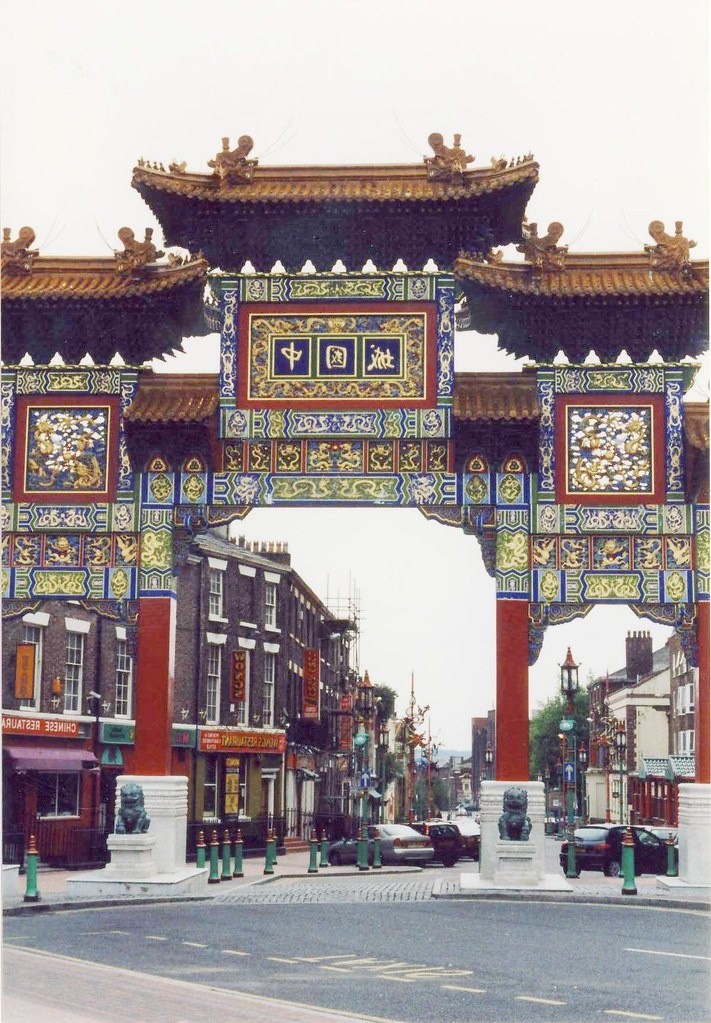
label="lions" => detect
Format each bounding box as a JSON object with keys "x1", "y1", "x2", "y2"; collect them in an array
[
  {"x1": 497, "y1": 786, "x2": 533, "y2": 841},
  {"x1": 116, "y1": 783, "x2": 151, "y2": 834}
]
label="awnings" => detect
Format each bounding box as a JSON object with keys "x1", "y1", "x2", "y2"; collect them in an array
[
  {"x1": 2, "y1": 746, "x2": 101, "y2": 776},
  {"x1": 303, "y1": 768, "x2": 321, "y2": 782},
  {"x1": 369, "y1": 790, "x2": 382, "y2": 799}
]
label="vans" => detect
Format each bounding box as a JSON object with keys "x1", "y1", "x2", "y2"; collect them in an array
[{"x1": 406, "y1": 823, "x2": 463, "y2": 867}]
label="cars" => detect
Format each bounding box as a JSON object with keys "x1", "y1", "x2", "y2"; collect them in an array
[
  {"x1": 642, "y1": 826, "x2": 680, "y2": 846},
  {"x1": 455, "y1": 803, "x2": 479, "y2": 818},
  {"x1": 327, "y1": 824, "x2": 435, "y2": 868},
  {"x1": 559, "y1": 824, "x2": 679, "y2": 877},
  {"x1": 442, "y1": 818, "x2": 480, "y2": 861}
]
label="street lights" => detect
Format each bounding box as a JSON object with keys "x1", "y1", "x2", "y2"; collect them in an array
[
  {"x1": 357, "y1": 670, "x2": 374, "y2": 871},
  {"x1": 557, "y1": 647, "x2": 582, "y2": 879}
]
